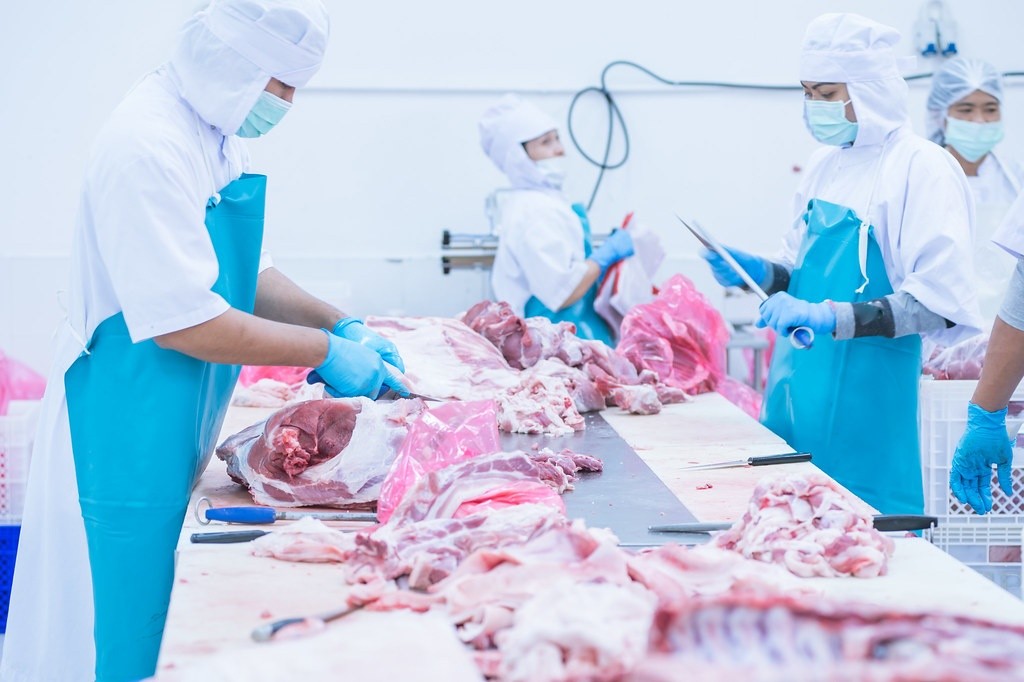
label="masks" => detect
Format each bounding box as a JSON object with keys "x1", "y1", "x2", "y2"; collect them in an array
[
  {"x1": 535, "y1": 158, "x2": 571, "y2": 193},
  {"x1": 801, "y1": 100, "x2": 858, "y2": 149},
  {"x1": 236, "y1": 90, "x2": 294, "y2": 139},
  {"x1": 943, "y1": 116, "x2": 1005, "y2": 164}
]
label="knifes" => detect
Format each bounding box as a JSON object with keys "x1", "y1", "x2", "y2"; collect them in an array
[
  {"x1": 682, "y1": 452, "x2": 815, "y2": 471},
  {"x1": 306, "y1": 369, "x2": 442, "y2": 404}
]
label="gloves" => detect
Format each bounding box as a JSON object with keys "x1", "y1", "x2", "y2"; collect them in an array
[
  {"x1": 587, "y1": 227, "x2": 634, "y2": 286},
  {"x1": 753, "y1": 291, "x2": 836, "y2": 338},
  {"x1": 949, "y1": 400, "x2": 1014, "y2": 516},
  {"x1": 702, "y1": 245, "x2": 771, "y2": 288},
  {"x1": 306, "y1": 316, "x2": 413, "y2": 401}
]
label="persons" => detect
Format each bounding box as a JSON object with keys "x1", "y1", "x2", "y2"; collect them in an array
[
  {"x1": 479, "y1": 93, "x2": 634, "y2": 350},
  {"x1": 703, "y1": 11, "x2": 1024, "y2": 539},
  {"x1": 0, "y1": 0, "x2": 411, "y2": 682}
]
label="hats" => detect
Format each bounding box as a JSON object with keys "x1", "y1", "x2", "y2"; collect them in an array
[
  {"x1": 925, "y1": 51, "x2": 1004, "y2": 146},
  {"x1": 795, "y1": 12, "x2": 913, "y2": 147},
  {"x1": 475, "y1": 91, "x2": 561, "y2": 193},
  {"x1": 164, "y1": 0, "x2": 334, "y2": 136}
]
